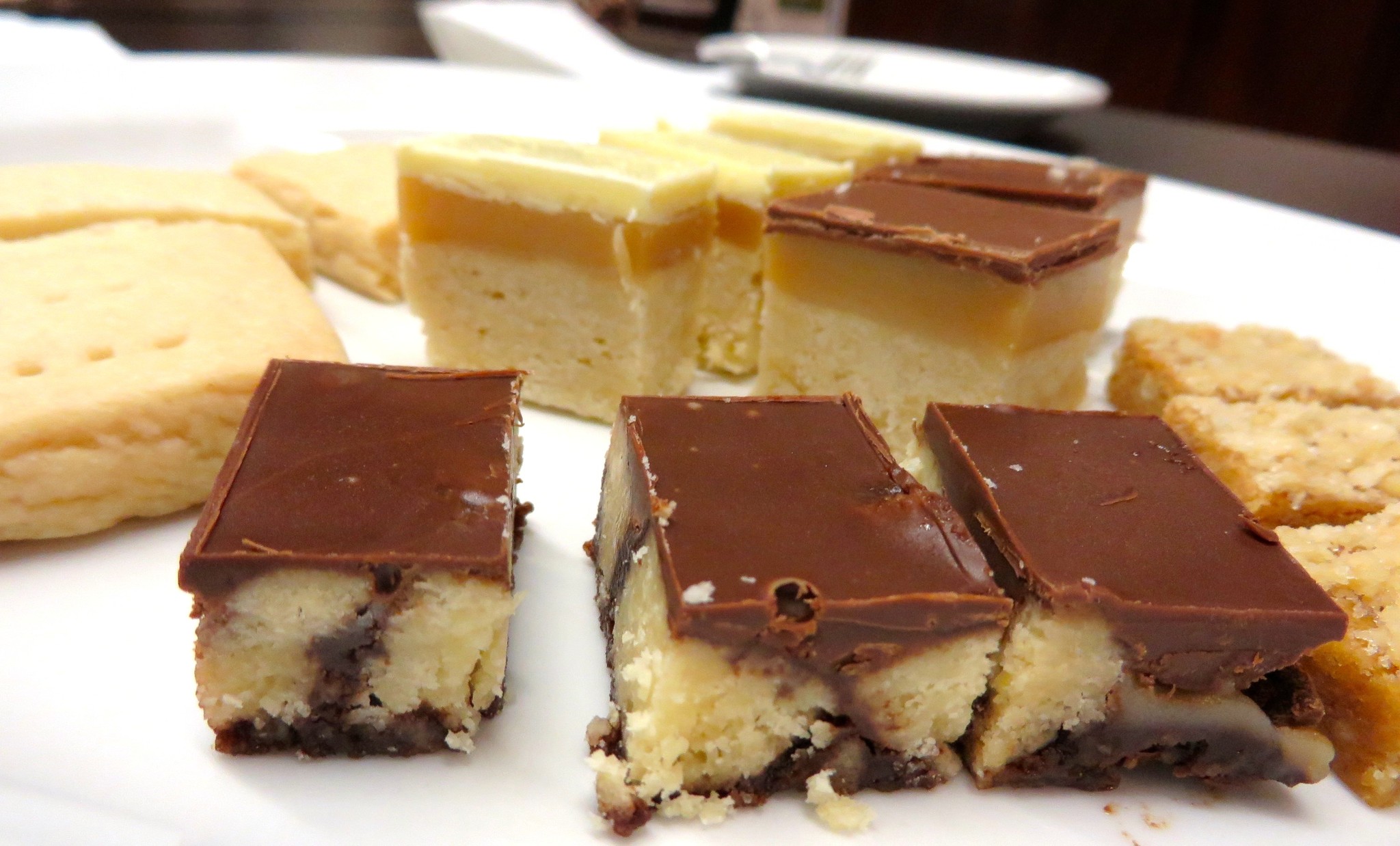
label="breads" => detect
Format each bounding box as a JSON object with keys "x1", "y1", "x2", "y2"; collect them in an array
[{"x1": 0, "y1": 146, "x2": 400, "y2": 542}]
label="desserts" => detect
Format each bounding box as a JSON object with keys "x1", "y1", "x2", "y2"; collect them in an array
[
  {"x1": 913, "y1": 400, "x2": 1349, "y2": 790},
  {"x1": 1106, "y1": 321, "x2": 1399, "y2": 810},
  {"x1": 584, "y1": 398, "x2": 1016, "y2": 838},
  {"x1": 758, "y1": 156, "x2": 1146, "y2": 470},
  {"x1": 393, "y1": 113, "x2": 922, "y2": 426},
  {"x1": 181, "y1": 360, "x2": 532, "y2": 759}
]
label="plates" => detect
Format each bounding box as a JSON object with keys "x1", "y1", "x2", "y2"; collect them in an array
[
  {"x1": 0, "y1": 52, "x2": 1397, "y2": 843},
  {"x1": 694, "y1": 30, "x2": 1113, "y2": 130}
]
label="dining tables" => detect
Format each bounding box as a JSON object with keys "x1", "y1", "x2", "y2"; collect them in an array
[{"x1": 1, "y1": 23, "x2": 1400, "y2": 236}]
